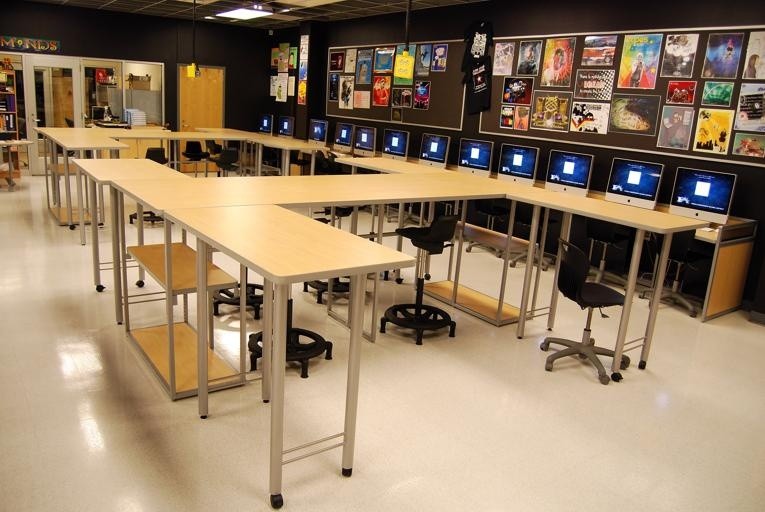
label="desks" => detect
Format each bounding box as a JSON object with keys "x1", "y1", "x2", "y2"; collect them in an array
[
  {"x1": 72, "y1": 158, "x2": 193, "y2": 291},
  {"x1": 334, "y1": 157, "x2": 558, "y2": 328},
  {"x1": 38, "y1": 127, "x2": 130, "y2": 230},
  {"x1": 0, "y1": 139, "x2": 34, "y2": 191},
  {"x1": 165, "y1": 206, "x2": 418, "y2": 511},
  {"x1": 195, "y1": 128, "x2": 272, "y2": 177},
  {"x1": 32, "y1": 126, "x2": 170, "y2": 227},
  {"x1": 170, "y1": 131, "x2": 276, "y2": 178},
  {"x1": 111, "y1": 169, "x2": 508, "y2": 403},
  {"x1": 246, "y1": 137, "x2": 331, "y2": 175},
  {"x1": 508, "y1": 191, "x2": 711, "y2": 382}
]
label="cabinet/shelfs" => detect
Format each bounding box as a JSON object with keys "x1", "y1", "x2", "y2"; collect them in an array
[{"x1": 0, "y1": 68, "x2": 21, "y2": 180}]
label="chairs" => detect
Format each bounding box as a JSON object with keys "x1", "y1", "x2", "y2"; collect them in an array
[
  {"x1": 315, "y1": 150, "x2": 328, "y2": 172},
  {"x1": 380, "y1": 214, "x2": 457, "y2": 344},
  {"x1": 206, "y1": 140, "x2": 225, "y2": 177},
  {"x1": 326, "y1": 150, "x2": 344, "y2": 175},
  {"x1": 182, "y1": 140, "x2": 209, "y2": 178},
  {"x1": 510, "y1": 202, "x2": 548, "y2": 271},
  {"x1": 216, "y1": 148, "x2": 240, "y2": 177},
  {"x1": 358, "y1": 167, "x2": 392, "y2": 216},
  {"x1": 388, "y1": 203, "x2": 425, "y2": 228},
  {"x1": 324, "y1": 205, "x2": 368, "y2": 229},
  {"x1": 303, "y1": 217, "x2": 349, "y2": 304},
  {"x1": 17, "y1": 117, "x2": 28, "y2": 168},
  {"x1": 248, "y1": 282, "x2": 333, "y2": 378},
  {"x1": 228, "y1": 140, "x2": 240, "y2": 150},
  {"x1": 214, "y1": 266, "x2": 264, "y2": 320},
  {"x1": 639, "y1": 229, "x2": 698, "y2": 320},
  {"x1": 586, "y1": 217, "x2": 629, "y2": 287},
  {"x1": 64, "y1": 118, "x2": 75, "y2": 159},
  {"x1": 129, "y1": 209, "x2": 163, "y2": 224},
  {"x1": 465, "y1": 198, "x2": 511, "y2": 259},
  {"x1": 145, "y1": 147, "x2": 168, "y2": 166},
  {"x1": 539, "y1": 237, "x2": 630, "y2": 384},
  {"x1": 440, "y1": 201, "x2": 461, "y2": 216},
  {"x1": 583, "y1": 230, "x2": 599, "y2": 262},
  {"x1": 313, "y1": 205, "x2": 364, "y2": 227},
  {"x1": 640, "y1": 243, "x2": 671, "y2": 289}
]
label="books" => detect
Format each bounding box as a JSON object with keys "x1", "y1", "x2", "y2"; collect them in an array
[
  {"x1": 0, "y1": 113, "x2": 14, "y2": 130},
  {"x1": 125, "y1": 108, "x2": 146, "y2": 126},
  {"x1": 0, "y1": 93, "x2": 15, "y2": 110}
]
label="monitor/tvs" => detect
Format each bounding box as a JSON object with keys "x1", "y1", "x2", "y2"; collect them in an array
[
  {"x1": 382, "y1": 129, "x2": 410, "y2": 161},
  {"x1": 260, "y1": 115, "x2": 271, "y2": 132},
  {"x1": 544, "y1": 149, "x2": 594, "y2": 197},
  {"x1": 308, "y1": 119, "x2": 328, "y2": 146},
  {"x1": 419, "y1": 134, "x2": 451, "y2": 168},
  {"x1": 605, "y1": 157, "x2": 664, "y2": 209},
  {"x1": 278, "y1": 116, "x2": 289, "y2": 134},
  {"x1": 458, "y1": 138, "x2": 494, "y2": 175},
  {"x1": 669, "y1": 167, "x2": 736, "y2": 224},
  {"x1": 498, "y1": 143, "x2": 540, "y2": 184},
  {"x1": 333, "y1": 122, "x2": 354, "y2": 154},
  {"x1": 354, "y1": 126, "x2": 376, "y2": 156}
]
